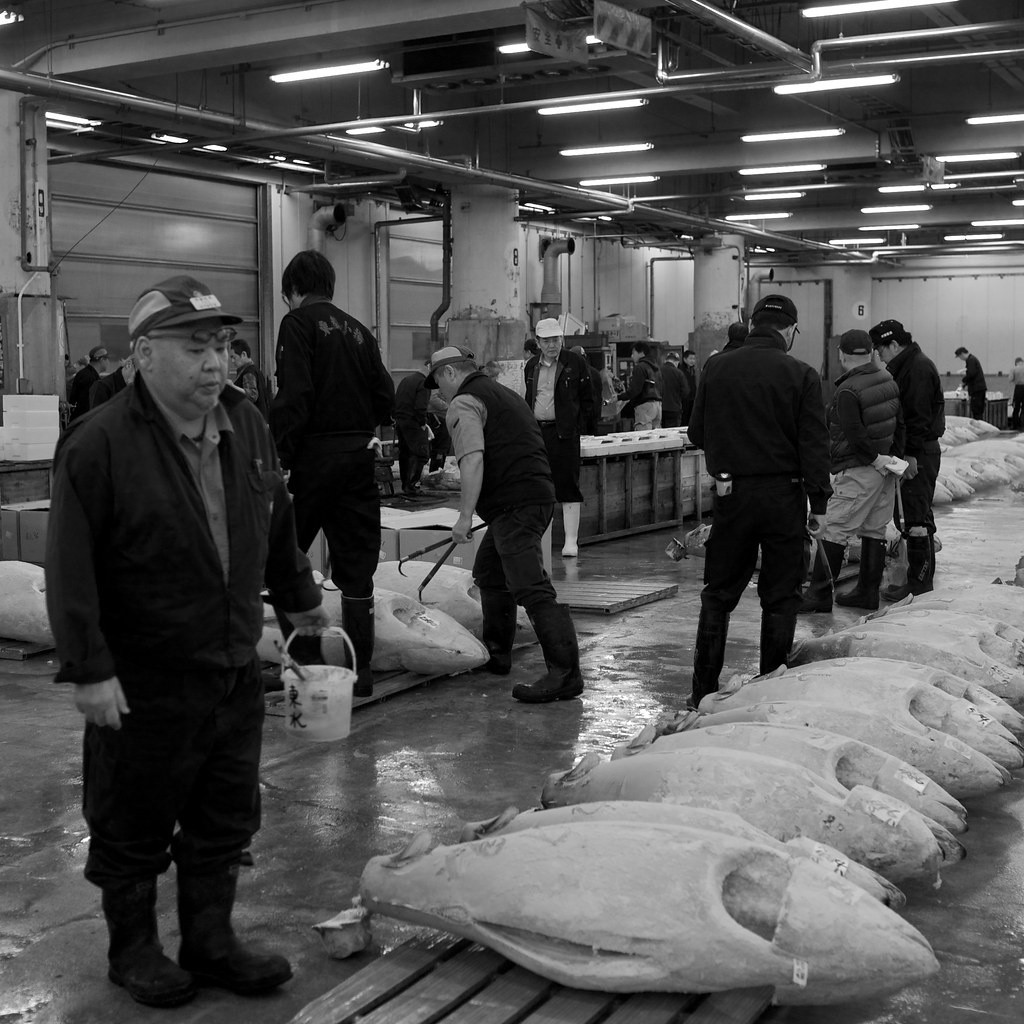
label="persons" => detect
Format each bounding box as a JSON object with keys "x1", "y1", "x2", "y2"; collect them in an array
[
  {"x1": 394, "y1": 358, "x2": 500, "y2": 501},
  {"x1": 722, "y1": 322, "x2": 749, "y2": 352},
  {"x1": 88, "y1": 354, "x2": 140, "y2": 410},
  {"x1": 70, "y1": 346, "x2": 110, "y2": 424},
  {"x1": 1008, "y1": 357, "x2": 1024, "y2": 433},
  {"x1": 44, "y1": 275, "x2": 330, "y2": 1010},
  {"x1": 678, "y1": 350, "x2": 696, "y2": 426},
  {"x1": 613, "y1": 341, "x2": 664, "y2": 431},
  {"x1": 686, "y1": 295, "x2": 835, "y2": 711},
  {"x1": 955, "y1": 347, "x2": 987, "y2": 420},
  {"x1": 64, "y1": 354, "x2": 77, "y2": 399},
  {"x1": 265, "y1": 250, "x2": 394, "y2": 697},
  {"x1": 422, "y1": 346, "x2": 584, "y2": 704},
  {"x1": 797, "y1": 329, "x2": 907, "y2": 615},
  {"x1": 224, "y1": 339, "x2": 274, "y2": 425},
  {"x1": 869, "y1": 319, "x2": 946, "y2": 603},
  {"x1": 660, "y1": 352, "x2": 690, "y2": 428},
  {"x1": 523, "y1": 317, "x2": 593, "y2": 557},
  {"x1": 523, "y1": 339, "x2": 603, "y2": 436}
]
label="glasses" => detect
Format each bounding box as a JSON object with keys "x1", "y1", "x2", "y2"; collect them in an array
[{"x1": 148, "y1": 327, "x2": 238, "y2": 344}]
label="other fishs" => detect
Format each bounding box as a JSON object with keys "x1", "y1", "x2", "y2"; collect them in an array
[
  {"x1": 1, "y1": 560, "x2": 54, "y2": 644},
  {"x1": 458, "y1": 585, "x2": 1023, "y2": 910},
  {"x1": 684, "y1": 517, "x2": 942, "y2": 571},
  {"x1": 255, "y1": 569, "x2": 490, "y2": 675},
  {"x1": 932, "y1": 415, "x2": 1024, "y2": 502},
  {"x1": 360, "y1": 819, "x2": 942, "y2": 1006},
  {"x1": 373, "y1": 561, "x2": 536, "y2": 644}
]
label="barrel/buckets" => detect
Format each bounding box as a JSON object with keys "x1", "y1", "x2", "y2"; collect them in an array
[{"x1": 279, "y1": 625, "x2": 358, "y2": 744}]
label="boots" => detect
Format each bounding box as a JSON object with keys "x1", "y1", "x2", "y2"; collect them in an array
[
  {"x1": 473, "y1": 589, "x2": 517, "y2": 675},
  {"x1": 512, "y1": 603, "x2": 584, "y2": 701},
  {"x1": 686, "y1": 608, "x2": 731, "y2": 712},
  {"x1": 882, "y1": 533, "x2": 936, "y2": 601},
  {"x1": 100, "y1": 882, "x2": 192, "y2": 1009},
  {"x1": 561, "y1": 502, "x2": 580, "y2": 556},
  {"x1": 797, "y1": 538, "x2": 849, "y2": 613},
  {"x1": 341, "y1": 594, "x2": 375, "y2": 697},
  {"x1": 174, "y1": 863, "x2": 294, "y2": 994},
  {"x1": 760, "y1": 613, "x2": 797, "y2": 675},
  {"x1": 835, "y1": 537, "x2": 885, "y2": 610}
]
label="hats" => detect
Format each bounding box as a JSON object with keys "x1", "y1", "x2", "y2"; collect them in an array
[
  {"x1": 424, "y1": 345, "x2": 477, "y2": 390},
  {"x1": 751, "y1": 294, "x2": 799, "y2": 322},
  {"x1": 955, "y1": 347, "x2": 965, "y2": 358},
  {"x1": 89, "y1": 346, "x2": 111, "y2": 358},
  {"x1": 1014, "y1": 358, "x2": 1022, "y2": 366},
  {"x1": 869, "y1": 319, "x2": 905, "y2": 350},
  {"x1": 127, "y1": 275, "x2": 243, "y2": 353},
  {"x1": 839, "y1": 329, "x2": 873, "y2": 355},
  {"x1": 536, "y1": 318, "x2": 564, "y2": 338}
]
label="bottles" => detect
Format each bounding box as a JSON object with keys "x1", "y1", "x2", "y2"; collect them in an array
[
  {"x1": 383, "y1": 442, "x2": 390, "y2": 457},
  {"x1": 715, "y1": 472, "x2": 733, "y2": 496}
]
